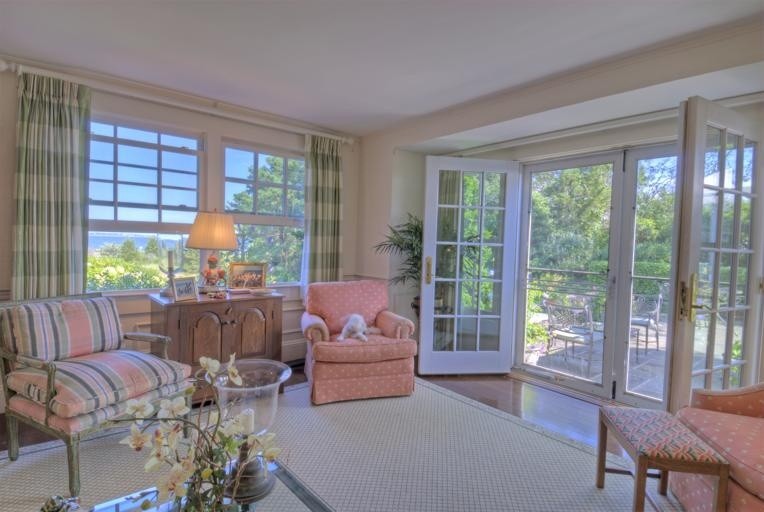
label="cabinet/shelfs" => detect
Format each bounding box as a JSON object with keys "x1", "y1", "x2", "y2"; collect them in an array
[{"x1": 148, "y1": 287, "x2": 286, "y2": 405}]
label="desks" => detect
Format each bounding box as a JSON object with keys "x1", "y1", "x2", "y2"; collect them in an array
[
  {"x1": 87, "y1": 459, "x2": 331, "y2": 511},
  {"x1": 571, "y1": 322, "x2": 640, "y2": 363}
]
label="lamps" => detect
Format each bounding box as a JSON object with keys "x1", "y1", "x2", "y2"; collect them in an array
[
  {"x1": 185, "y1": 207, "x2": 239, "y2": 293},
  {"x1": 155, "y1": 228, "x2": 185, "y2": 296},
  {"x1": 204, "y1": 357, "x2": 293, "y2": 505}
]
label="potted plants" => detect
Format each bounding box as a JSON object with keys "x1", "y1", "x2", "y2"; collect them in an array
[
  {"x1": 522, "y1": 281, "x2": 545, "y2": 365},
  {"x1": 370, "y1": 208, "x2": 484, "y2": 323}
]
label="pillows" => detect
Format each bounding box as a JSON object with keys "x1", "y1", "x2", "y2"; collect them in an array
[{"x1": 11, "y1": 296, "x2": 123, "y2": 369}]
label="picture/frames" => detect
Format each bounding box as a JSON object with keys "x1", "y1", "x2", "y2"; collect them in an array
[
  {"x1": 171, "y1": 276, "x2": 197, "y2": 302},
  {"x1": 228, "y1": 262, "x2": 267, "y2": 289}
]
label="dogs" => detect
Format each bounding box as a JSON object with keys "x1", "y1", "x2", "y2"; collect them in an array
[{"x1": 336, "y1": 313, "x2": 382, "y2": 342}]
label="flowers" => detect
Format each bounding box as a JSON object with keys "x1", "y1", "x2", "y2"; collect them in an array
[{"x1": 93, "y1": 349, "x2": 282, "y2": 511}]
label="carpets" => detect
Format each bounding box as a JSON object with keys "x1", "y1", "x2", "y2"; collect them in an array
[{"x1": 0, "y1": 376, "x2": 684, "y2": 511}]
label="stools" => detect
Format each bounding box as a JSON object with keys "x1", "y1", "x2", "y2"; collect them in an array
[{"x1": 596, "y1": 403, "x2": 731, "y2": 512}]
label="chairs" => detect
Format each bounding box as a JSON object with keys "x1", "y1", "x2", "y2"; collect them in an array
[
  {"x1": 667, "y1": 380, "x2": 764, "y2": 512},
  {"x1": 630, "y1": 293, "x2": 662, "y2": 355},
  {"x1": 0, "y1": 292, "x2": 198, "y2": 499},
  {"x1": 541, "y1": 300, "x2": 603, "y2": 378},
  {"x1": 301, "y1": 279, "x2": 419, "y2": 405}
]
